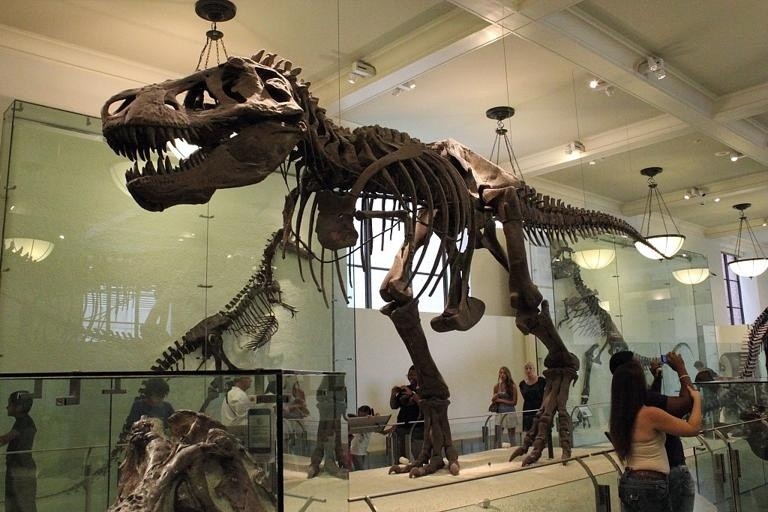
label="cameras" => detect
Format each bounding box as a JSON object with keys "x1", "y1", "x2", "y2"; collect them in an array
[
  {"x1": 660, "y1": 355, "x2": 668, "y2": 363},
  {"x1": 396, "y1": 387, "x2": 406, "y2": 392}
]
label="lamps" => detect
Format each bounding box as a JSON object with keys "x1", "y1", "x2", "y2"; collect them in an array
[
  {"x1": 727, "y1": 151, "x2": 751, "y2": 165},
  {"x1": 633, "y1": 55, "x2": 665, "y2": 81},
  {"x1": 727, "y1": 202, "x2": 768, "y2": 280},
  {"x1": 3, "y1": 237, "x2": 57, "y2": 265},
  {"x1": 347, "y1": 61, "x2": 376, "y2": 84},
  {"x1": 564, "y1": 140, "x2": 585, "y2": 156},
  {"x1": 631, "y1": 166, "x2": 686, "y2": 264},
  {"x1": 571, "y1": 248, "x2": 615, "y2": 269},
  {"x1": 683, "y1": 185, "x2": 707, "y2": 202},
  {"x1": 167, "y1": 2, "x2": 246, "y2": 163},
  {"x1": 480, "y1": 105, "x2": 526, "y2": 226},
  {"x1": 586, "y1": 77, "x2": 618, "y2": 98},
  {"x1": 109, "y1": 159, "x2": 158, "y2": 199},
  {"x1": 392, "y1": 79, "x2": 417, "y2": 98},
  {"x1": 672, "y1": 265, "x2": 710, "y2": 286}
]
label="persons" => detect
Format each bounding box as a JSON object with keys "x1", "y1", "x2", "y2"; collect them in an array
[
  {"x1": 519, "y1": 361, "x2": 546, "y2": 445},
  {"x1": 126, "y1": 378, "x2": 175, "y2": 432},
  {"x1": 349, "y1": 405, "x2": 397, "y2": 471},
  {"x1": 493, "y1": 367, "x2": 517, "y2": 448},
  {"x1": 390, "y1": 365, "x2": 424, "y2": 465},
  {"x1": 609, "y1": 350, "x2": 721, "y2": 512},
  {"x1": 0, "y1": 390, "x2": 37, "y2": 512},
  {"x1": 221, "y1": 377, "x2": 309, "y2": 456}
]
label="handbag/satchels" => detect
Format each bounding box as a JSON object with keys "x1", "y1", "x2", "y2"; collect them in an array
[{"x1": 488, "y1": 400, "x2": 498, "y2": 413}]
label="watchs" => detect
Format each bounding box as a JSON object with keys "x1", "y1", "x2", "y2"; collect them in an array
[{"x1": 409, "y1": 391, "x2": 416, "y2": 398}]
